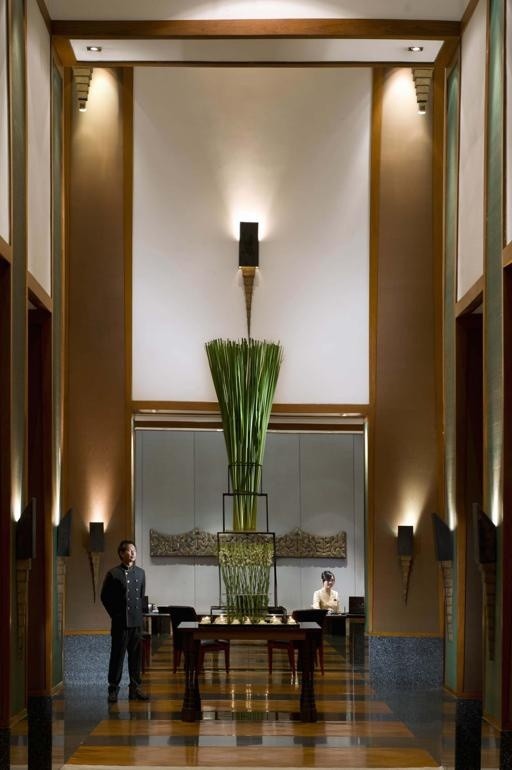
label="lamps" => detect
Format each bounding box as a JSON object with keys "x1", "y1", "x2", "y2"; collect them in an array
[
  {"x1": 15, "y1": 494, "x2": 38, "y2": 663},
  {"x1": 236, "y1": 220, "x2": 259, "y2": 340},
  {"x1": 57, "y1": 506, "x2": 106, "y2": 656},
  {"x1": 397, "y1": 524, "x2": 416, "y2": 609},
  {"x1": 71, "y1": 65, "x2": 94, "y2": 113},
  {"x1": 430, "y1": 501, "x2": 497, "y2": 662},
  {"x1": 410, "y1": 64, "x2": 435, "y2": 115}
]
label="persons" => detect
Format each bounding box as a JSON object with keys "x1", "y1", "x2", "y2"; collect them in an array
[
  {"x1": 100, "y1": 540, "x2": 149, "y2": 703},
  {"x1": 312, "y1": 571, "x2": 341, "y2": 612}
]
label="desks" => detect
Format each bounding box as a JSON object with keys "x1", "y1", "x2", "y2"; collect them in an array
[{"x1": 170, "y1": 619, "x2": 322, "y2": 725}]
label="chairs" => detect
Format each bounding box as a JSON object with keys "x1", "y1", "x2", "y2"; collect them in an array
[
  {"x1": 267, "y1": 608, "x2": 330, "y2": 677},
  {"x1": 166, "y1": 606, "x2": 232, "y2": 673}
]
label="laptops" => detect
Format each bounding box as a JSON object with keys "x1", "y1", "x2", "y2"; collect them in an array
[{"x1": 330, "y1": 596, "x2": 364, "y2": 615}]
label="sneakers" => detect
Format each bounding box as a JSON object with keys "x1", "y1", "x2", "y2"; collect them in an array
[
  {"x1": 107, "y1": 684, "x2": 120, "y2": 702},
  {"x1": 127, "y1": 685, "x2": 150, "y2": 701}
]
